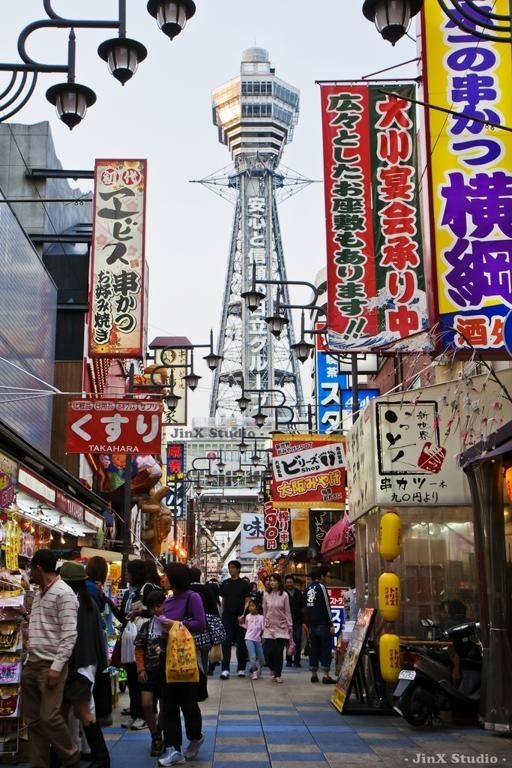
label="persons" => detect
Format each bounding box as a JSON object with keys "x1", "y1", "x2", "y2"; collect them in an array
[
  {"x1": 20, "y1": 548, "x2": 83, "y2": 768},
  {"x1": 82, "y1": 555, "x2": 113, "y2": 728},
  {"x1": 104, "y1": 558, "x2": 336, "y2": 766},
  {"x1": 57, "y1": 560, "x2": 112, "y2": 767}
]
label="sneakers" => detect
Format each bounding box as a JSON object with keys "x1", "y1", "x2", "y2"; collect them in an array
[
  {"x1": 311, "y1": 676, "x2": 336, "y2": 684},
  {"x1": 271, "y1": 673, "x2": 283, "y2": 683},
  {"x1": 150, "y1": 733, "x2": 205, "y2": 766},
  {"x1": 221, "y1": 668, "x2": 260, "y2": 681},
  {"x1": 286, "y1": 661, "x2": 302, "y2": 668},
  {"x1": 120, "y1": 708, "x2": 148, "y2": 729}
]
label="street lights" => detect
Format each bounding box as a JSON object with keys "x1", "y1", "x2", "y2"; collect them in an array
[
  {"x1": 358, "y1": 0, "x2": 432, "y2": 43},
  {"x1": 237, "y1": 261, "x2": 368, "y2": 430},
  {"x1": 169, "y1": 450, "x2": 226, "y2": 563},
  {"x1": 122, "y1": 327, "x2": 222, "y2": 549},
  {"x1": 226, "y1": 425, "x2": 273, "y2": 507},
  {"x1": 0, "y1": 0, "x2": 194, "y2": 133}
]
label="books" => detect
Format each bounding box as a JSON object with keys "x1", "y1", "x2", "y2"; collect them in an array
[{"x1": 0, "y1": 593, "x2": 26, "y2": 718}]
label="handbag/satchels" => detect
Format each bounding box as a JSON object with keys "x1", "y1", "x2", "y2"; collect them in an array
[{"x1": 179, "y1": 613, "x2": 226, "y2": 651}]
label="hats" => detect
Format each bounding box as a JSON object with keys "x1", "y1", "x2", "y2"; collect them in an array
[{"x1": 60, "y1": 561, "x2": 89, "y2": 582}]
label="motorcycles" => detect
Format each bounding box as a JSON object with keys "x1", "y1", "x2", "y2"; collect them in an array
[{"x1": 391, "y1": 612, "x2": 488, "y2": 724}]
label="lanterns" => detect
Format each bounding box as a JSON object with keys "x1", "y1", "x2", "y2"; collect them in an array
[
  {"x1": 378, "y1": 509, "x2": 402, "y2": 564},
  {"x1": 379, "y1": 629, "x2": 402, "y2": 691},
  {"x1": 377, "y1": 570, "x2": 401, "y2": 623},
  {"x1": 505, "y1": 467, "x2": 512, "y2": 503}
]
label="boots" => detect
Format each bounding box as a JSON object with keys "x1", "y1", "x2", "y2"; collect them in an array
[{"x1": 83, "y1": 720, "x2": 111, "y2": 767}]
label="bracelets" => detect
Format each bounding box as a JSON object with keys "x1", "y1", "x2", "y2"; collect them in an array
[{"x1": 23, "y1": 611, "x2": 31, "y2": 620}]
